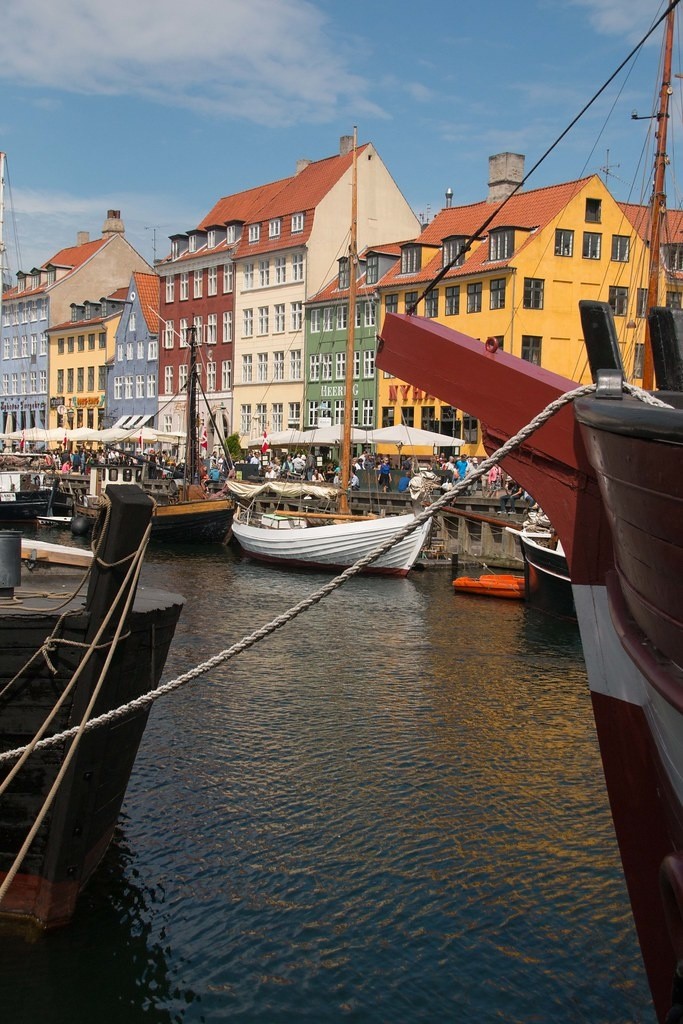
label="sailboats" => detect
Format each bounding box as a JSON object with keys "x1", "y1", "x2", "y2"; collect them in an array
[
  {"x1": 74, "y1": 313, "x2": 240, "y2": 546},
  {"x1": 423, "y1": 0, "x2": 679, "y2": 622},
  {"x1": 225, "y1": 123, "x2": 434, "y2": 577}
]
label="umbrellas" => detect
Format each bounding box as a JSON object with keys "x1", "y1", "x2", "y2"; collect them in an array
[
  {"x1": 0, "y1": 426, "x2": 184, "y2": 455},
  {"x1": 249, "y1": 424, "x2": 466, "y2": 470}
]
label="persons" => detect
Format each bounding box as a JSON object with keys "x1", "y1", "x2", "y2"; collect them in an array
[{"x1": 0, "y1": 441, "x2": 545, "y2": 515}]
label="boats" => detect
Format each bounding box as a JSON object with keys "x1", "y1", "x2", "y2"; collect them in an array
[
  {"x1": 36, "y1": 514, "x2": 73, "y2": 529},
  {"x1": 0, "y1": 452, "x2": 73, "y2": 521},
  {"x1": 0, "y1": 482, "x2": 184, "y2": 936},
  {"x1": 451, "y1": 574, "x2": 526, "y2": 599},
  {"x1": 377, "y1": 0, "x2": 681, "y2": 1024}
]
